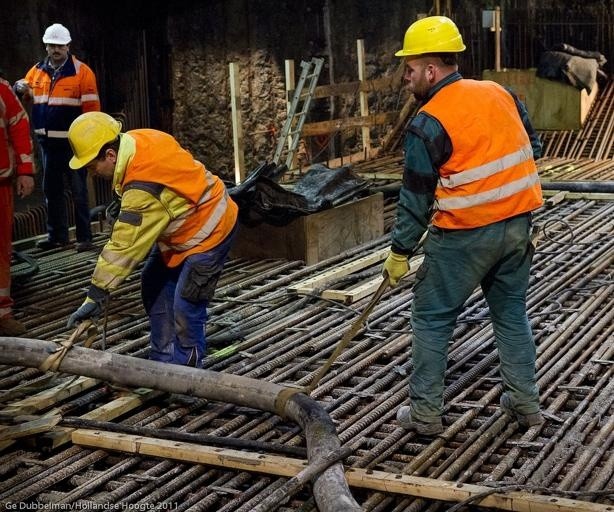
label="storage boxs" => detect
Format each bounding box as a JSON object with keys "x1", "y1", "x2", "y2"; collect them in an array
[{"x1": 227, "y1": 190, "x2": 385, "y2": 267}]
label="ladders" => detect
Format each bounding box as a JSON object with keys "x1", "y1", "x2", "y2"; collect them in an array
[{"x1": 273, "y1": 56, "x2": 324, "y2": 170}]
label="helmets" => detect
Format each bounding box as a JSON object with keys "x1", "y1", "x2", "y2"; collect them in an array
[
  {"x1": 42, "y1": 23, "x2": 72, "y2": 46},
  {"x1": 68, "y1": 111, "x2": 124, "y2": 169},
  {"x1": 394, "y1": 16, "x2": 468, "y2": 59}
]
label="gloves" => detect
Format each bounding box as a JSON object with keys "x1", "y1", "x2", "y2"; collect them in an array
[
  {"x1": 65, "y1": 294, "x2": 102, "y2": 330},
  {"x1": 383, "y1": 252, "x2": 409, "y2": 288}
]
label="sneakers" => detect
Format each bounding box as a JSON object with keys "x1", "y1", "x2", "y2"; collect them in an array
[
  {"x1": 76, "y1": 236, "x2": 94, "y2": 251},
  {"x1": 396, "y1": 406, "x2": 444, "y2": 436},
  {"x1": 0, "y1": 315, "x2": 27, "y2": 336},
  {"x1": 171, "y1": 391, "x2": 198, "y2": 405},
  {"x1": 37, "y1": 237, "x2": 70, "y2": 249},
  {"x1": 500, "y1": 391, "x2": 545, "y2": 426}
]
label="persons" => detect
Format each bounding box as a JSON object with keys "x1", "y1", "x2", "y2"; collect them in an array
[
  {"x1": 15, "y1": 23, "x2": 102, "y2": 253},
  {"x1": 382, "y1": 16, "x2": 547, "y2": 436},
  {"x1": 67, "y1": 109, "x2": 241, "y2": 411},
  {"x1": 0, "y1": 76, "x2": 38, "y2": 336}
]
